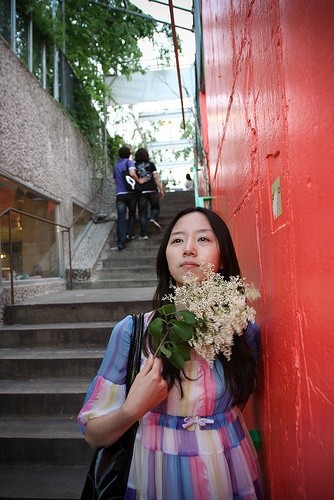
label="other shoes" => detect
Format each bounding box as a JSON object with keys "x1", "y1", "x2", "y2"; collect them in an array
[
  {"x1": 126, "y1": 234, "x2": 136, "y2": 240},
  {"x1": 138, "y1": 232, "x2": 149, "y2": 240},
  {"x1": 117, "y1": 242, "x2": 125, "y2": 249},
  {"x1": 149, "y1": 218, "x2": 162, "y2": 232}
]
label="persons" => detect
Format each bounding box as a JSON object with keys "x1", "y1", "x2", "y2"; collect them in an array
[
  {"x1": 185, "y1": 173, "x2": 193, "y2": 191},
  {"x1": 76, "y1": 207, "x2": 267, "y2": 500},
  {"x1": 132, "y1": 148, "x2": 164, "y2": 240},
  {"x1": 112, "y1": 146, "x2": 147, "y2": 250}
]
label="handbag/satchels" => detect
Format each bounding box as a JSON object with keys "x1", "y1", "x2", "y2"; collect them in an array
[
  {"x1": 122, "y1": 161, "x2": 145, "y2": 193},
  {"x1": 81, "y1": 312, "x2": 144, "y2": 500}
]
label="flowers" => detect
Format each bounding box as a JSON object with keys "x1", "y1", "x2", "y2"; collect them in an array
[{"x1": 145, "y1": 257, "x2": 261, "y2": 369}]
